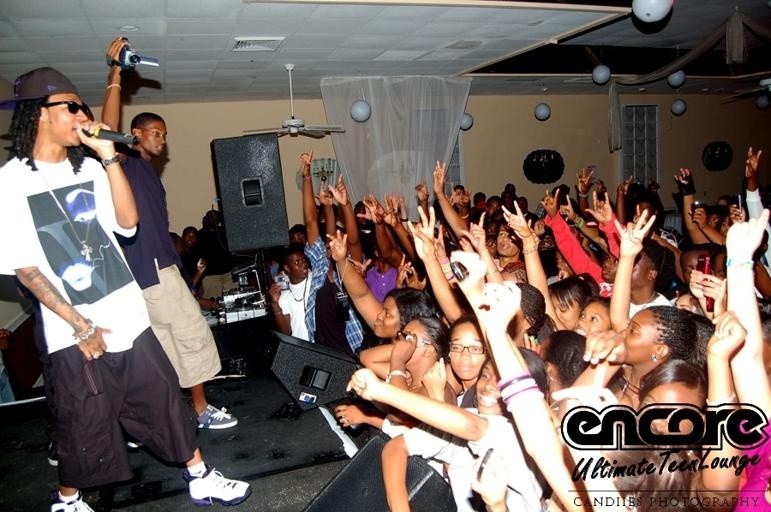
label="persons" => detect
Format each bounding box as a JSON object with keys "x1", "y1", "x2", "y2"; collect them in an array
[
  {"x1": 453, "y1": 185, "x2": 470, "y2": 213},
  {"x1": 433, "y1": 160, "x2": 498, "y2": 258},
  {"x1": 349, "y1": 192, "x2": 405, "y2": 304},
  {"x1": 432, "y1": 224, "x2": 458, "y2": 287},
  {"x1": 679, "y1": 244, "x2": 710, "y2": 287},
  {"x1": 745, "y1": 147, "x2": 771, "y2": 275},
  {"x1": 474, "y1": 171, "x2": 521, "y2": 283},
  {"x1": 45, "y1": 36, "x2": 239, "y2": 467},
  {"x1": 671, "y1": 166, "x2": 746, "y2": 249},
  {"x1": 674, "y1": 293, "x2": 705, "y2": 317},
  {"x1": 269, "y1": 150, "x2": 330, "y2": 343},
  {"x1": 399, "y1": 197, "x2": 408, "y2": 232},
  {"x1": 314, "y1": 172, "x2": 364, "y2": 343},
  {"x1": 688, "y1": 253, "x2": 771, "y2": 386},
  {"x1": 0, "y1": 65, "x2": 251, "y2": 512},
  {"x1": 502, "y1": 199, "x2": 611, "y2": 336},
  {"x1": 460, "y1": 190, "x2": 471, "y2": 232},
  {"x1": 472, "y1": 449, "x2": 566, "y2": 512},
  {"x1": 628, "y1": 240, "x2": 677, "y2": 320},
  {"x1": 571, "y1": 312, "x2": 747, "y2": 512},
  {"x1": 289, "y1": 223, "x2": 307, "y2": 245},
  {"x1": 354, "y1": 200, "x2": 377, "y2": 259},
  {"x1": 512, "y1": 270, "x2": 599, "y2": 330},
  {"x1": 575, "y1": 169, "x2": 595, "y2": 220},
  {"x1": 414, "y1": 179, "x2": 431, "y2": 222},
  {"x1": 560, "y1": 194, "x2": 608, "y2": 255},
  {"x1": 540, "y1": 188, "x2": 621, "y2": 297},
  {"x1": 523, "y1": 332, "x2": 584, "y2": 411},
  {"x1": 617, "y1": 176, "x2": 682, "y2": 279},
  {"x1": 335, "y1": 403, "x2": 448, "y2": 486},
  {"x1": 346, "y1": 347, "x2": 554, "y2": 512},
  {"x1": 521, "y1": 183, "x2": 575, "y2": 251},
  {"x1": 609, "y1": 210, "x2": 705, "y2": 333},
  {"x1": 450, "y1": 249, "x2": 690, "y2": 512},
  {"x1": 326, "y1": 230, "x2": 437, "y2": 343},
  {"x1": 318, "y1": 204, "x2": 345, "y2": 240},
  {"x1": 359, "y1": 315, "x2": 457, "y2": 444},
  {"x1": 726, "y1": 208, "x2": 771, "y2": 512},
  {"x1": 422, "y1": 311, "x2": 489, "y2": 411},
  {"x1": 407, "y1": 206, "x2": 557, "y2": 355},
  {"x1": 165, "y1": 208, "x2": 231, "y2": 314},
  {"x1": 381, "y1": 192, "x2": 416, "y2": 263},
  {"x1": 710, "y1": 245, "x2": 728, "y2": 281}
]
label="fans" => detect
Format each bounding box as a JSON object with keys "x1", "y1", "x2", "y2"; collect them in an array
[
  {"x1": 720, "y1": 75, "x2": 771, "y2": 102},
  {"x1": 240, "y1": 61, "x2": 345, "y2": 141}
]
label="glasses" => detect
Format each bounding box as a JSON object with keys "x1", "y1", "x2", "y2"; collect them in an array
[
  {"x1": 45, "y1": 102, "x2": 89, "y2": 116},
  {"x1": 448, "y1": 344, "x2": 485, "y2": 356}
]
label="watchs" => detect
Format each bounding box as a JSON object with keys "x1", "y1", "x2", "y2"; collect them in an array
[
  {"x1": 102, "y1": 153, "x2": 121, "y2": 165},
  {"x1": 386, "y1": 370, "x2": 407, "y2": 383}
]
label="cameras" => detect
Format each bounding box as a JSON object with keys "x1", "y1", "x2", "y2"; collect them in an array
[
  {"x1": 120, "y1": 37, "x2": 161, "y2": 69},
  {"x1": 335, "y1": 290, "x2": 348, "y2": 308}
]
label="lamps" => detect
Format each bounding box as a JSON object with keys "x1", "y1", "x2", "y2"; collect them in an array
[
  {"x1": 671, "y1": 92, "x2": 688, "y2": 115},
  {"x1": 458, "y1": 111, "x2": 476, "y2": 133},
  {"x1": 667, "y1": 41, "x2": 686, "y2": 87},
  {"x1": 349, "y1": 73, "x2": 371, "y2": 125},
  {"x1": 632, "y1": 0, "x2": 674, "y2": 23},
  {"x1": 589, "y1": 33, "x2": 611, "y2": 85},
  {"x1": 533, "y1": 78, "x2": 552, "y2": 121},
  {"x1": 757, "y1": 98, "x2": 771, "y2": 112}
]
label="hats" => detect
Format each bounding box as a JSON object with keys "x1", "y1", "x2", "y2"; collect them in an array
[{"x1": 0, "y1": 68, "x2": 80, "y2": 110}]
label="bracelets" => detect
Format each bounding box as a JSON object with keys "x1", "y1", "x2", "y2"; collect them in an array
[
  {"x1": 498, "y1": 371, "x2": 545, "y2": 412},
  {"x1": 439, "y1": 257, "x2": 455, "y2": 281},
  {"x1": 303, "y1": 173, "x2": 312, "y2": 182},
  {"x1": 726, "y1": 257, "x2": 755, "y2": 266},
  {"x1": 706, "y1": 394, "x2": 737, "y2": 411},
  {"x1": 335, "y1": 259, "x2": 348, "y2": 288},
  {"x1": 107, "y1": 83, "x2": 122, "y2": 89},
  {"x1": 73, "y1": 318, "x2": 97, "y2": 341},
  {"x1": 576, "y1": 217, "x2": 584, "y2": 228},
  {"x1": 400, "y1": 217, "x2": 409, "y2": 222},
  {"x1": 462, "y1": 213, "x2": 470, "y2": 219},
  {"x1": 522, "y1": 243, "x2": 539, "y2": 254}
]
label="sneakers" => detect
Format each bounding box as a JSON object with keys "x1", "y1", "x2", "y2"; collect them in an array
[
  {"x1": 182, "y1": 466, "x2": 253, "y2": 506},
  {"x1": 50, "y1": 491, "x2": 95, "y2": 512},
  {"x1": 196, "y1": 405, "x2": 238, "y2": 430}
]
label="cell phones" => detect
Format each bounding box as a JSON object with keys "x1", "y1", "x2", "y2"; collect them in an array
[
  {"x1": 733, "y1": 192, "x2": 742, "y2": 211},
  {"x1": 691, "y1": 201, "x2": 700, "y2": 216},
  {"x1": 697, "y1": 254, "x2": 711, "y2": 282}
]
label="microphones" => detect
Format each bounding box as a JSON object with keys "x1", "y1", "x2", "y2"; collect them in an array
[{"x1": 80, "y1": 127, "x2": 139, "y2": 146}]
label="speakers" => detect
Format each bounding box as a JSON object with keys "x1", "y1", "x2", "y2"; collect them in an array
[
  {"x1": 211, "y1": 133, "x2": 290, "y2": 255},
  {"x1": 266, "y1": 328, "x2": 357, "y2": 412},
  {"x1": 304, "y1": 430, "x2": 457, "y2": 512}
]
